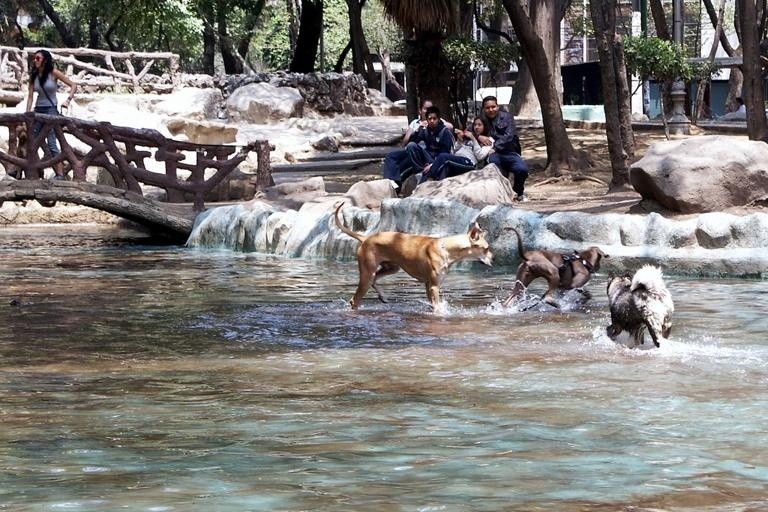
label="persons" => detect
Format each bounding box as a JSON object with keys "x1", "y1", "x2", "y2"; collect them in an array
[
  {"x1": 382, "y1": 96, "x2": 528, "y2": 204},
  {"x1": 718, "y1": 97, "x2": 747, "y2": 122},
  {"x1": 26, "y1": 50, "x2": 77, "y2": 181}
]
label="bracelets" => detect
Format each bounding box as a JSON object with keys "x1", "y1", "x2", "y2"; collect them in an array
[{"x1": 67, "y1": 96, "x2": 72, "y2": 102}]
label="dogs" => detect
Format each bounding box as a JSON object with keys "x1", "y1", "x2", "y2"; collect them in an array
[
  {"x1": 603, "y1": 261, "x2": 677, "y2": 346},
  {"x1": 499, "y1": 226, "x2": 610, "y2": 313},
  {"x1": 334, "y1": 201, "x2": 497, "y2": 312}
]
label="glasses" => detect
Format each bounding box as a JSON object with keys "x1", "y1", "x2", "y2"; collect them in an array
[{"x1": 32, "y1": 55, "x2": 44, "y2": 62}]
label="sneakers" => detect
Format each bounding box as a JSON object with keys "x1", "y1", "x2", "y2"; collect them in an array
[{"x1": 516, "y1": 194, "x2": 531, "y2": 204}]
